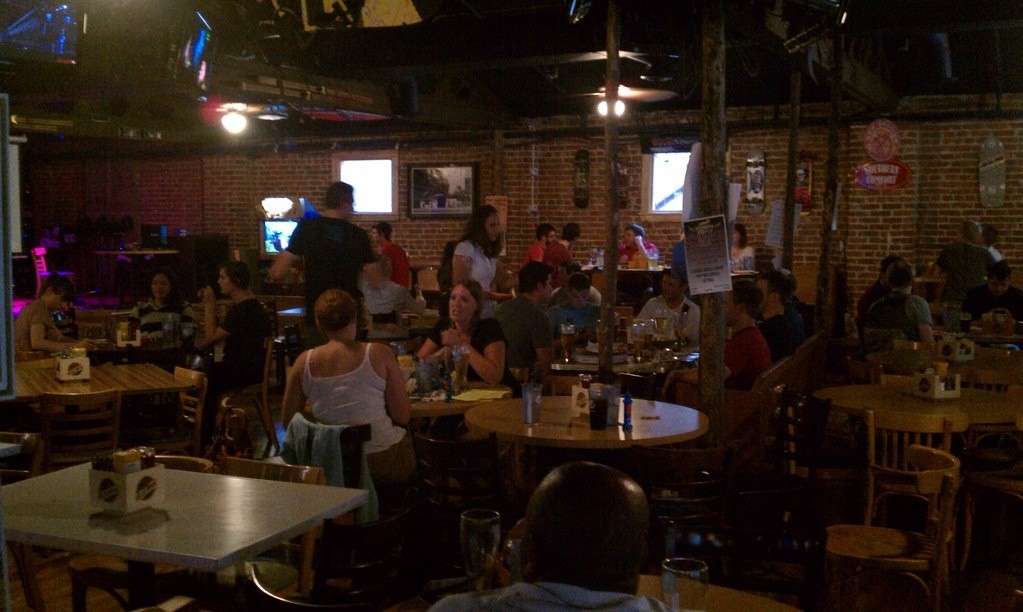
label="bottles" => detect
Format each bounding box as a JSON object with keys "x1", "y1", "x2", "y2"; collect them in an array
[
  {"x1": 94, "y1": 446, "x2": 155, "y2": 471},
  {"x1": 414, "y1": 289, "x2": 426, "y2": 310}
]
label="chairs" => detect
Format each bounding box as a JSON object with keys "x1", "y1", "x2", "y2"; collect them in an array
[{"x1": 1, "y1": 289, "x2": 1023, "y2": 612}]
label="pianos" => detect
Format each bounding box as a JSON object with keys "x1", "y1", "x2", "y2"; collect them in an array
[{"x1": 93, "y1": 244, "x2": 181, "y2": 310}]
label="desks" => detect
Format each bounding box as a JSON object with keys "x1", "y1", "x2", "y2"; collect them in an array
[
  {"x1": 581, "y1": 263, "x2": 765, "y2": 309},
  {"x1": 4, "y1": 356, "x2": 192, "y2": 466},
  {"x1": 865, "y1": 347, "x2": 1022, "y2": 387},
  {"x1": 0, "y1": 463, "x2": 369, "y2": 612},
  {"x1": 806, "y1": 380, "x2": 1022, "y2": 431},
  {"x1": 95, "y1": 249, "x2": 174, "y2": 303},
  {"x1": 464, "y1": 393, "x2": 707, "y2": 484},
  {"x1": 633, "y1": 574, "x2": 804, "y2": 612},
  {"x1": 373, "y1": 358, "x2": 514, "y2": 416},
  {"x1": 86, "y1": 323, "x2": 231, "y2": 357},
  {"x1": 537, "y1": 342, "x2": 696, "y2": 391}
]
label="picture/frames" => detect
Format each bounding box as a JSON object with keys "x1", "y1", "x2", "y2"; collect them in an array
[{"x1": 405, "y1": 159, "x2": 478, "y2": 220}]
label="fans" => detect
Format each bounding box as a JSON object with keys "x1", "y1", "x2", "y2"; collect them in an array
[{"x1": 566, "y1": 74, "x2": 679, "y2": 105}]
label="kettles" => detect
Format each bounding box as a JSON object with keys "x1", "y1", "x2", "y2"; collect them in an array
[{"x1": 653, "y1": 308, "x2": 669, "y2": 333}]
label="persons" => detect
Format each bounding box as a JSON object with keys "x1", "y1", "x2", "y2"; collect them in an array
[
  {"x1": 523, "y1": 222, "x2": 581, "y2": 289},
  {"x1": 618, "y1": 224, "x2": 662, "y2": 265},
  {"x1": 496, "y1": 261, "x2": 557, "y2": 381},
  {"x1": 931, "y1": 220, "x2": 1003, "y2": 301},
  {"x1": 427, "y1": 461, "x2": 674, "y2": 612},
  {"x1": 635, "y1": 269, "x2": 699, "y2": 337},
  {"x1": 961, "y1": 261, "x2": 1023, "y2": 322},
  {"x1": 417, "y1": 278, "x2": 523, "y2": 398},
  {"x1": 361, "y1": 221, "x2": 410, "y2": 312},
  {"x1": 200, "y1": 260, "x2": 266, "y2": 450},
  {"x1": 128, "y1": 264, "x2": 194, "y2": 367},
  {"x1": 280, "y1": 288, "x2": 417, "y2": 485},
  {"x1": 755, "y1": 268, "x2": 805, "y2": 364},
  {"x1": 856, "y1": 255, "x2": 937, "y2": 343},
  {"x1": 731, "y1": 224, "x2": 756, "y2": 262},
  {"x1": 547, "y1": 261, "x2": 602, "y2": 333},
  {"x1": 663, "y1": 279, "x2": 771, "y2": 406},
  {"x1": 452, "y1": 204, "x2": 513, "y2": 320},
  {"x1": 273, "y1": 181, "x2": 377, "y2": 347},
  {"x1": 14, "y1": 272, "x2": 96, "y2": 354}
]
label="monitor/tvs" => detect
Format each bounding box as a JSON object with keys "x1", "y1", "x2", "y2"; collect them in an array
[{"x1": 259, "y1": 219, "x2": 300, "y2": 259}]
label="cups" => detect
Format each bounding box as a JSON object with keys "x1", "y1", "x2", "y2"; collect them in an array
[
  {"x1": 520, "y1": 382, "x2": 544, "y2": 424},
  {"x1": 588, "y1": 383, "x2": 608, "y2": 430},
  {"x1": 452, "y1": 346, "x2": 470, "y2": 388},
  {"x1": 959, "y1": 313, "x2": 972, "y2": 336},
  {"x1": 460, "y1": 508, "x2": 500, "y2": 583},
  {"x1": 659, "y1": 558, "x2": 710, "y2": 612},
  {"x1": 590, "y1": 249, "x2": 605, "y2": 269},
  {"x1": 560, "y1": 323, "x2": 575, "y2": 362},
  {"x1": 648, "y1": 251, "x2": 657, "y2": 269}
]
